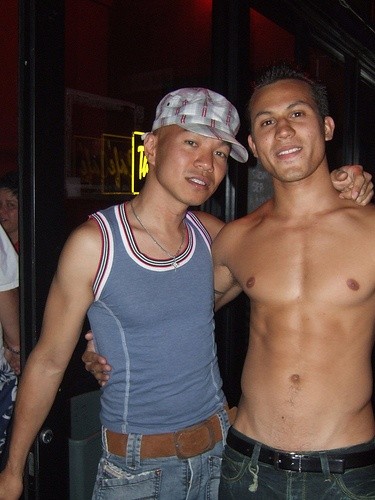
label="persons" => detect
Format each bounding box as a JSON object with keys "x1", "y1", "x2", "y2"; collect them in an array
[
  {"x1": 81, "y1": 66, "x2": 375, "y2": 499},
  {"x1": 0, "y1": 225, "x2": 20, "y2": 468},
  {"x1": 0, "y1": 172, "x2": 21, "y2": 255},
  {"x1": 0, "y1": 86, "x2": 374, "y2": 500}
]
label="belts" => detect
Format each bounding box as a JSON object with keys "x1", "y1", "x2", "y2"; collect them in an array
[
  {"x1": 227, "y1": 430, "x2": 375, "y2": 473},
  {"x1": 103, "y1": 401, "x2": 238, "y2": 459}
]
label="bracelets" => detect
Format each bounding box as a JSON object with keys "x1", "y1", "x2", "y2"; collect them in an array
[{"x1": 3, "y1": 341, "x2": 21, "y2": 355}]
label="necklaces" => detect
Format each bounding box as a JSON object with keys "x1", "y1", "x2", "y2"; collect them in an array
[{"x1": 130, "y1": 199, "x2": 185, "y2": 272}]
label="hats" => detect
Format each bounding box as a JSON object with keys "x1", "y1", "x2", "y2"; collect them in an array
[{"x1": 151, "y1": 89, "x2": 250, "y2": 164}]
